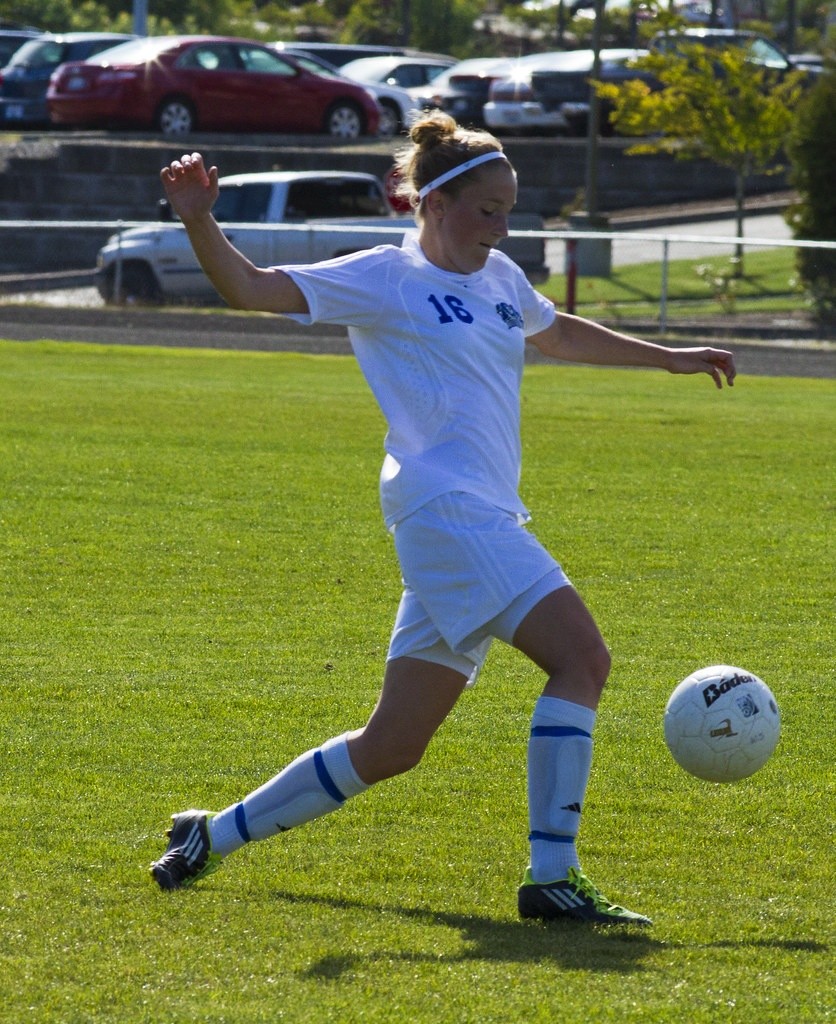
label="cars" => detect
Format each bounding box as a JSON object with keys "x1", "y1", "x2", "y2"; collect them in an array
[
  {"x1": 275, "y1": 36, "x2": 648, "y2": 141},
  {"x1": 0, "y1": 34, "x2": 141, "y2": 132},
  {"x1": 48, "y1": 34, "x2": 379, "y2": 144}
]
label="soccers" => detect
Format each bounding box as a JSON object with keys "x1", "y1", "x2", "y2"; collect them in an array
[{"x1": 663, "y1": 664, "x2": 781, "y2": 783}]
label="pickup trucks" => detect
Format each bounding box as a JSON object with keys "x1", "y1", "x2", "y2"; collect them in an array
[
  {"x1": 533, "y1": 27, "x2": 802, "y2": 141},
  {"x1": 95, "y1": 169, "x2": 550, "y2": 310}
]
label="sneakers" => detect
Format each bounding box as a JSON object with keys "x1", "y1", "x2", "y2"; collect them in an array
[
  {"x1": 516, "y1": 866, "x2": 654, "y2": 930},
  {"x1": 148, "y1": 809, "x2": 224, "y2": 893}
]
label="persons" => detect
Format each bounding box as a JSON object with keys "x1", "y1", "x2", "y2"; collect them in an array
[{"x1": 146, "y1": 104, "x2": 740, "y2": 920}]
